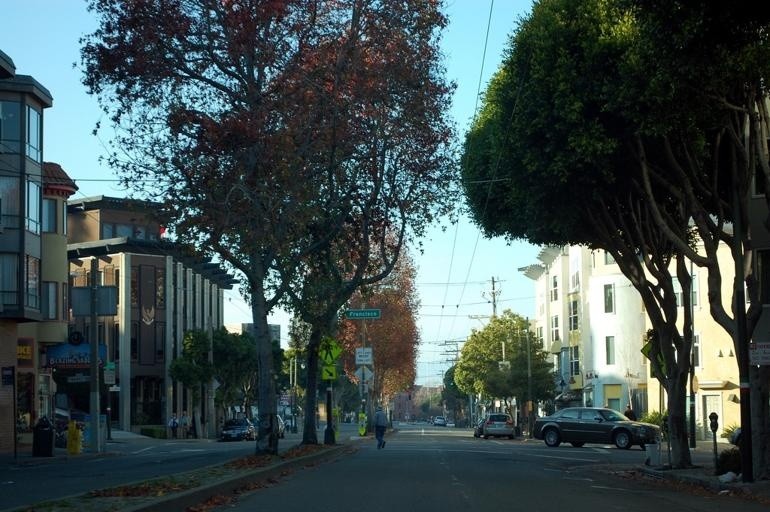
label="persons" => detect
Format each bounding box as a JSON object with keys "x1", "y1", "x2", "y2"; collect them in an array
[
  {"x1": 168, "y1": 410, "x2": 177, "y2": 439},
  {"x1": 178, "y1": 408, "x2": 193, "y2": 438},
  {"x1": 624, "y1": 403, "x2": 638, "y2": 422},
  {"x1": 370, "y1": 402, "x2": 388, "y2": 450}
]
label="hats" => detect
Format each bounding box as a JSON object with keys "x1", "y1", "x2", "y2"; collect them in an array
[{"x1": 377, "y1": 405, "x2": 383, "y2": 410}]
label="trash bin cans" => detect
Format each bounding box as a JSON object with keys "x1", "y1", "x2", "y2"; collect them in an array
[
  {"x1": 645, "y1": 445, "x2": 660, "y2": 467},
  {"x1": 33, "y1": 416, "x2": 56, "y2": 456}
]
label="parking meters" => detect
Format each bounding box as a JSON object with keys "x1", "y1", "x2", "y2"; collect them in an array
[
  {"x1": 661, "y1": 419, "x2": 672, "y2": 466},
  {"x1": 709, "y1": 414, "x2": 718, "y2": 463}
]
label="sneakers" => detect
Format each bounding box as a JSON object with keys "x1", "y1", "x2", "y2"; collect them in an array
[{"x1": 377, "y1": 440, "x2": 386, "y2": 449}]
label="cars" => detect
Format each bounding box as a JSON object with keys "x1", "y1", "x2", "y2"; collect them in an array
[
  {"x1": 219, "y1": 418, "x2": 256, "y2": 441},
  {"x1": 434, "y1": 416, "x2": 447, "y2": 426},
  {"x1": 475, "y1": 413, "x2": 516, "y2": 440},
  {"x1": 532, "y1": 407, "x2": 660, "y2": 450},
  {"x1": 255, "y1": 415, "x2": 285, "y2": 438}
]
